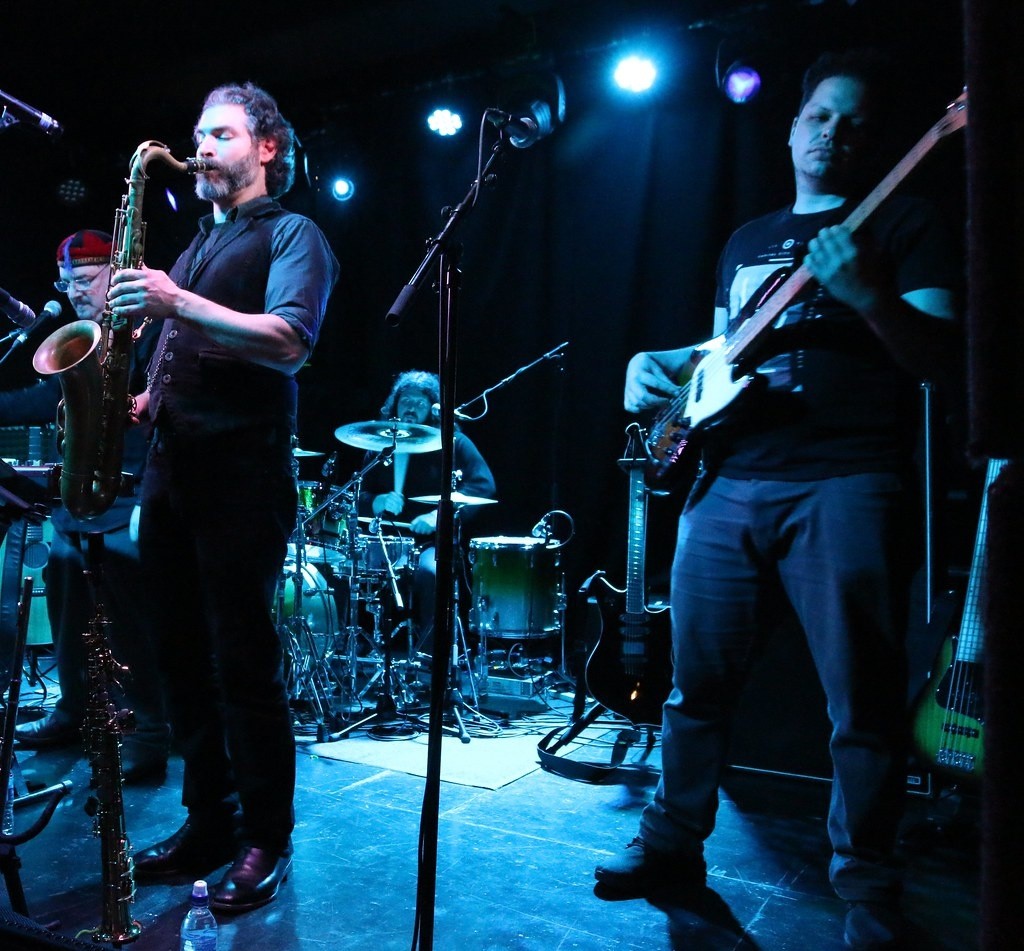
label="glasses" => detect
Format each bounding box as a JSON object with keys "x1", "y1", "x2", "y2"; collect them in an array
[{"x1": 54, "y1": 262, "x2": 110, "y2": 293}]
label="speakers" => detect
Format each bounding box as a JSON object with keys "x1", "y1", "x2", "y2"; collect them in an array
[{"x1": 720, "y1": 367, "x2": 977, "y2": 794}]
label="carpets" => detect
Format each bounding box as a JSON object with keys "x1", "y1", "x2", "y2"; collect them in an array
[{"x1": 296, "y1": 729, "x2": 614, "y2": 792}]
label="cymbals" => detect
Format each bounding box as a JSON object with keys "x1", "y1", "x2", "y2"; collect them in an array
[
  {"x1": 291, "y1": 447, "x2": 324, "y2": 458},
  {"x1": 334, "y1": 420, "x2": 456, "y2": 453},
  {"x1": 407, "y1": 493, "x2": 499, "y2": 507}
]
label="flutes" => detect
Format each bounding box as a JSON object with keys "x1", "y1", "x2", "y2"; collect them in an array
[{"x1": 0, "y1": 577, "x2": 34, "y2": 829}]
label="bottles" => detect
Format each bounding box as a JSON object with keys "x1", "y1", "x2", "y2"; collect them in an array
[
  {"x1": 1, "y1": 768, "x2": 14, "y2": 835},
  {"x1": 180, "y1": 880, "x2": 218, "y2": 951}
]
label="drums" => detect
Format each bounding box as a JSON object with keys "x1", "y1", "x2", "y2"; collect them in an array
[
  {"x1": 331, "y1": 535, "x2": 416, "y2": 579},
  {"x1": 287, "y1": 480, "x2": 357, "y2": 564},
  {"x1": 271, "y1": 564, "x2": 340, "y2": 699},
  {"x1": 468, "y1": 536, "x2": 566, "y2": 640}
]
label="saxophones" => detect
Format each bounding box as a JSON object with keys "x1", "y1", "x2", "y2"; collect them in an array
[
  {"x1": 32, "y1": 140, "x2": 216, "y2": 520},
  {"x1": 75, "y1": 570, "x2": 144, "y2": 943}
]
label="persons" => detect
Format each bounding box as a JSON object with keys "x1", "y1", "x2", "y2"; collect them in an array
[
  {"x1": 107, "y1": 82, "x2": 339, "y2": 912},
  {"x1": 596, "y1": 52, "x2": 966, "y2": 951},
  {"x1": 355, "y1": 370, "x2": 496, "y2": 652},
  {"x1": 0, "y1": 227, "x2": 172, "y2": 785}
]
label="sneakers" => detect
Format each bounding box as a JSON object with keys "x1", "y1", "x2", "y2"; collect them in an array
[
  {"x1": 595, "y1": 836, "x2": 707, "y2": 894},
  {"x1": 843, "y1": 898, "x2": 903, "y2": 951}
]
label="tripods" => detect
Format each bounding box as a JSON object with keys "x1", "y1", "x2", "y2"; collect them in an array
[{"x1": 277, "y1": 138, "x2": 508, "y2": 745}]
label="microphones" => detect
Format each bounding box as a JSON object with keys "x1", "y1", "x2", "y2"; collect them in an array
[
  {"x1": 0, "y1": 288, "x2": 35, "y2": 329},
  {"x1": 487, "y1": 108, "x2": 539, "y2": 148},
  {"x1": 432, "y1": 403, "x2": 471, "y2": 421},
  {"x1": 368, "y1": 513, "x2": 383, "y2": 533},
  {"x1": 12, "y1": 301, "x2": 62, "y2": 348},
  {"x1": 0, "y1": 90, "x2": 64, "y2": 137},
  {"x1": 321, "y1": 453, "x2": 337, "y2": 476},
  {"x1": 532, "y1": 513, "x2": 552, "y2": 537}
]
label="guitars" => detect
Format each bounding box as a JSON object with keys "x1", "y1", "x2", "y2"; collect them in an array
[
  {"x1": 583, "y1": 422, "x2": 675, "y2": 730},
  {"x1": 903, "y1": 459, "x2": 1011, "y2": 779},
  {"x1": 643, "y1": 86, "x2": 968, "y2": 492}
]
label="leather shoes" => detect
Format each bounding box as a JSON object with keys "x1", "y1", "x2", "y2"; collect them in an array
[
  {"x1": 14, "y1": 712, "x2": 70, "y2": 744},
  {"x1": 212, "y1": 844, "x2": 292, "y2": 911},
  {"x1": 132, "y1": 819, "x2": 246, "y2": 875},
  {"x1": 118, "y1": 757, "x2": 169, "y2": 788}
]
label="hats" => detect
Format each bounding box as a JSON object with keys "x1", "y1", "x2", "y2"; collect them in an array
[{"x1": 56, "y1": 230, "x2": 117, "y2": 268}]
